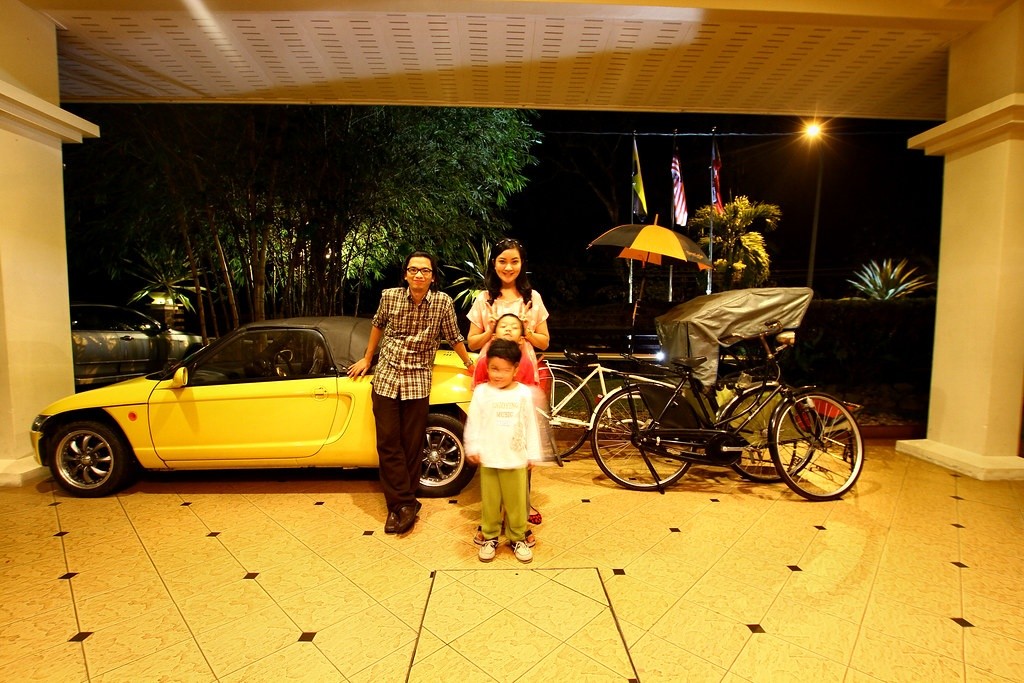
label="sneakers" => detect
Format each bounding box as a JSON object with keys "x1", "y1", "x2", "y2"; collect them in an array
[
  {"x1": 478, "y1": 540, "x2": 500, "y2": 563},
  {"x1": 511, "y1": 540, "x2": 533, "y2": 564}
]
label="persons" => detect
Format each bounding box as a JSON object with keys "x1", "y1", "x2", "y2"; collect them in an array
[
  {"x1": 347, "y1": 252, "x2": 475, "y2": 535},
  {"x1": 464, "y1": 237, "x2": 550, "y2": 563}
]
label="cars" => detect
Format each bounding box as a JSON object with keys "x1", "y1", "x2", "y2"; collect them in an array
[
  {"x1": 29, "y1": 317, "x2": 485, "y2": 498},
  {"x1": 69, "y1": 305, "x2": 206, "y2": 393}
]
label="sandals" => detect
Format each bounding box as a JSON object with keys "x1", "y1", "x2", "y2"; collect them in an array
[
  {"x1": 473, "y1": 525, "x2": 485, "y2": 545},
  {"x1": 524, "y1": 529, "x2": 536, "y2": 547}
]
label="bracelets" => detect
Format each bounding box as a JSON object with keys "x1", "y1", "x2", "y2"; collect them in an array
[
  {"x1": 464, "y1": 358, "x2": 473, "y2": 367},
  {"x1": 524, "y1": 328, "x2": 532, "y2": 340}
]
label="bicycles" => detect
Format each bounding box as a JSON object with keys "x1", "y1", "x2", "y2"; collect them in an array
[{"x1": 531, "y1": 320, "x2": 865, "y2": 502}]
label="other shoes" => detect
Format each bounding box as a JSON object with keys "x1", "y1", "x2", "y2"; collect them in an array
[{"x1": 528, "y1": 505, "x2": 542, "y2": 525}]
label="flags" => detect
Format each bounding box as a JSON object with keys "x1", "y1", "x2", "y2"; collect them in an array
[
  {"x1": 632, "y1": 138, "x2": 648, "y2": 223},
  {"x1": 671, "y1": 145, "x2": 688, "y2": 227},
  {"x1": 710, "y1": 141, "x2": 725, "y2": 213}
]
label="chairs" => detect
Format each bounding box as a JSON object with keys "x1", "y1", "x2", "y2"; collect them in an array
[{"x1": 308, "y1": 344, "x2": 328, "y2": 375}]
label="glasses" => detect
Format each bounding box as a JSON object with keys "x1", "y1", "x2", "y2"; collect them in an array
[{"x1": 407, "y1": 266, "x2": 433, "y2": 275}]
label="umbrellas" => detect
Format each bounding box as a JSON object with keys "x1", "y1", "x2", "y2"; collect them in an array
[{"x1": 583, "y1": 214, "x2": 716, "y2": 303}]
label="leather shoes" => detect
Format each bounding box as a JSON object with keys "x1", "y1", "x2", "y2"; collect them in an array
[{"x1": 384, "y1": 498, "x2": 422, "y2": 534}]
